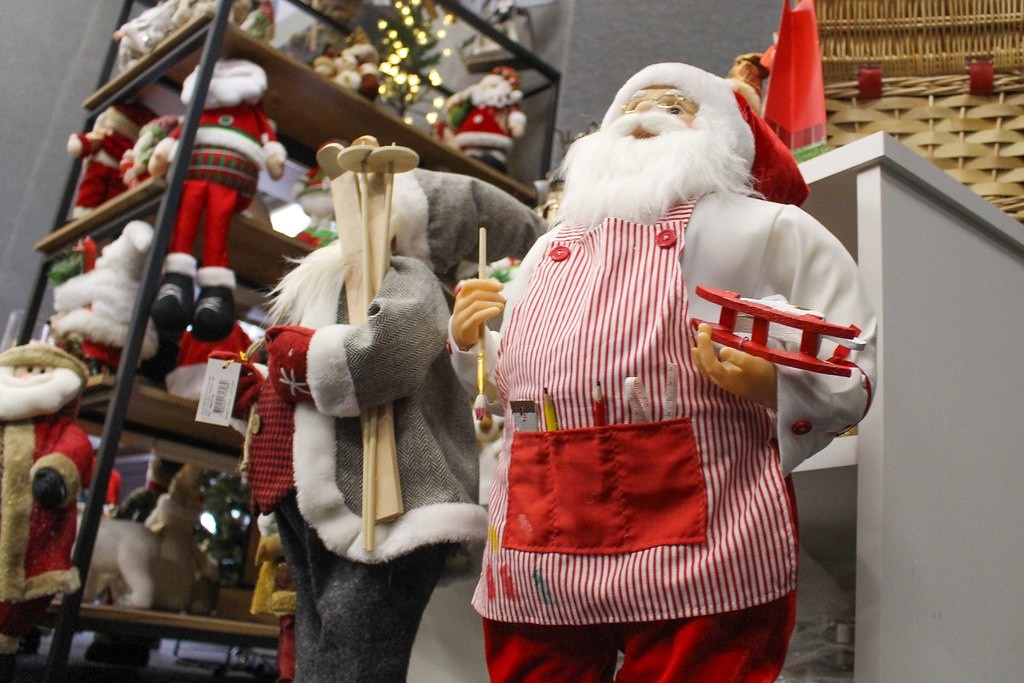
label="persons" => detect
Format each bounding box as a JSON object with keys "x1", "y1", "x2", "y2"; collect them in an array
[
  {"x1": 0, "y1": 341, "x2": 93, "y2": 683},
  {"x1": 113, "y1": 457, "x2": 185, "y2": 523},
  {"x1": 67, "y1": 57, "x2": 349, "y2": 343},
  {"x1": 446, "y1": 62, "x2": 878, "y2": 683},
  {"x1": 208, "y1": 167, "x2": 554, "y2": 683},
  {"x1": 454, "y1": 66, "x2": 527, "y2": 173}
]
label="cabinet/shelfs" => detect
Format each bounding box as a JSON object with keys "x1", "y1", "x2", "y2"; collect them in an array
[
  {"x1": 404, "y1": 130, "x2": 1024, "y2": 681},
  {"x1": 0, "y1": 1, "x2": 563, "y2": 681}
]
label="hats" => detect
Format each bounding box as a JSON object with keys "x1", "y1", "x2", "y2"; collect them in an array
[
  {"x1": 600, "y1": 63, "x2": 811, "y2": 206},
  {"x1": 389, "y1": 167, "x2": 549, "y2": 279}
]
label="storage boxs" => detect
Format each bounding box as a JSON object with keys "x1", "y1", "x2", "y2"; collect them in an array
[{"x1": 824, "y1": 69, "x2": 1024, "y2": 224}]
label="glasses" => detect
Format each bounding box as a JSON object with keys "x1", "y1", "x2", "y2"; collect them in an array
[{"x1": 624, "y1": 89, "x2": 697, "y2": 115}]
